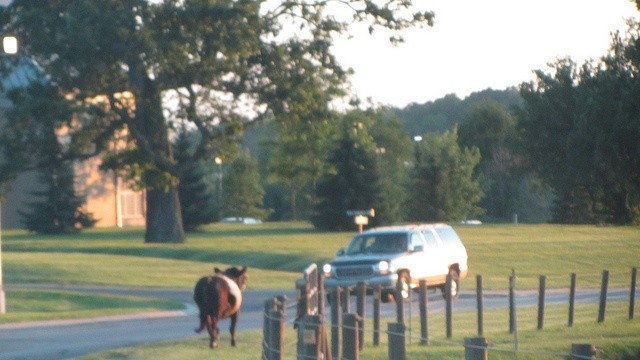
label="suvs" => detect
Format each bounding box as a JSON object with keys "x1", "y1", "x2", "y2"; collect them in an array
[{"x1": 323, "y1": 222, "x2": 469, "y2": 313}]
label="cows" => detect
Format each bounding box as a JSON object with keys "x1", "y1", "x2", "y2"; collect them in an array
[{"x1": 194, "y1": 265, "x2": 248, "y2": 350}]
